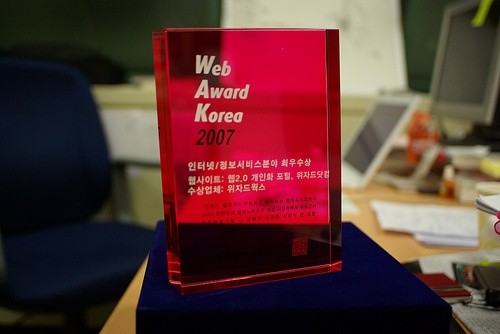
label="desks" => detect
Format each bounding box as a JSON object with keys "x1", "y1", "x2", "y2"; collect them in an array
[{"x1": 98, "y1": 177, "x2": 500, "y2": 333}]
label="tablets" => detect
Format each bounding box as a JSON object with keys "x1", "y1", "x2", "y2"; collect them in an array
[{"x1": 341, "y1": 94, "x2": 422, "y2": 192}]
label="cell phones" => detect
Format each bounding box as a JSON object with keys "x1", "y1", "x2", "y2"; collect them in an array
[{"x1": 473, "y1": 265, "x2": 500, "y2": 308}]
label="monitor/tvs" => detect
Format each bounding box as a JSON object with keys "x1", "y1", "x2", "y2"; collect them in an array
[{"x1": 427, "y1": 0, "x2": 500, "y2": 126}]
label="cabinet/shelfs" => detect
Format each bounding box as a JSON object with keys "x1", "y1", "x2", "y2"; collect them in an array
[{"x1": 94, "y1": 85, "x2": 428, "y2": 224}]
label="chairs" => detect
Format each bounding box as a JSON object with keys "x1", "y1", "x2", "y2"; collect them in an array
[{"x1": 0, "y1": 56, "x2": 154, "y2": 331}]
